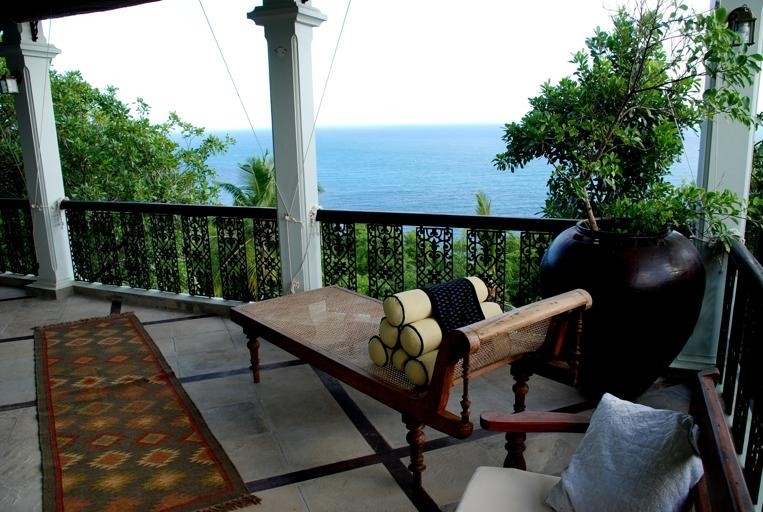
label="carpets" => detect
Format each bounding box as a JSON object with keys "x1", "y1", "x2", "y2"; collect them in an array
[{"x1": 31, "y1": 310, "x2": 262, "y2": 512}]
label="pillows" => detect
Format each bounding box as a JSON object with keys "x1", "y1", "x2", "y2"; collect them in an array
[
  {"x1": 368, "y1": 276, "x2": 503, "y2": 388},
  {"x1": 545, "y1": 393, "x2": 705, "y2": 512}
]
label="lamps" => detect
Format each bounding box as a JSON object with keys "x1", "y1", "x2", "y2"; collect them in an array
[{"x1": 0, "y1": 70, "x2": 22, "y2": 95}]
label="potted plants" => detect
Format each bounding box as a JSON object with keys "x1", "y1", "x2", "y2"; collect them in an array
[{"x1": 492, "y1": 0, "x2": 763, "y2": 407}]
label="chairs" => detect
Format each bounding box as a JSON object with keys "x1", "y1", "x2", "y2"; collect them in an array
[
  {"x1": 454, "y1": 367, "x2": 755, "y2": 512},
  {"x1": 230, "y1": 285, "x2": 593, "y2": 488}
]
